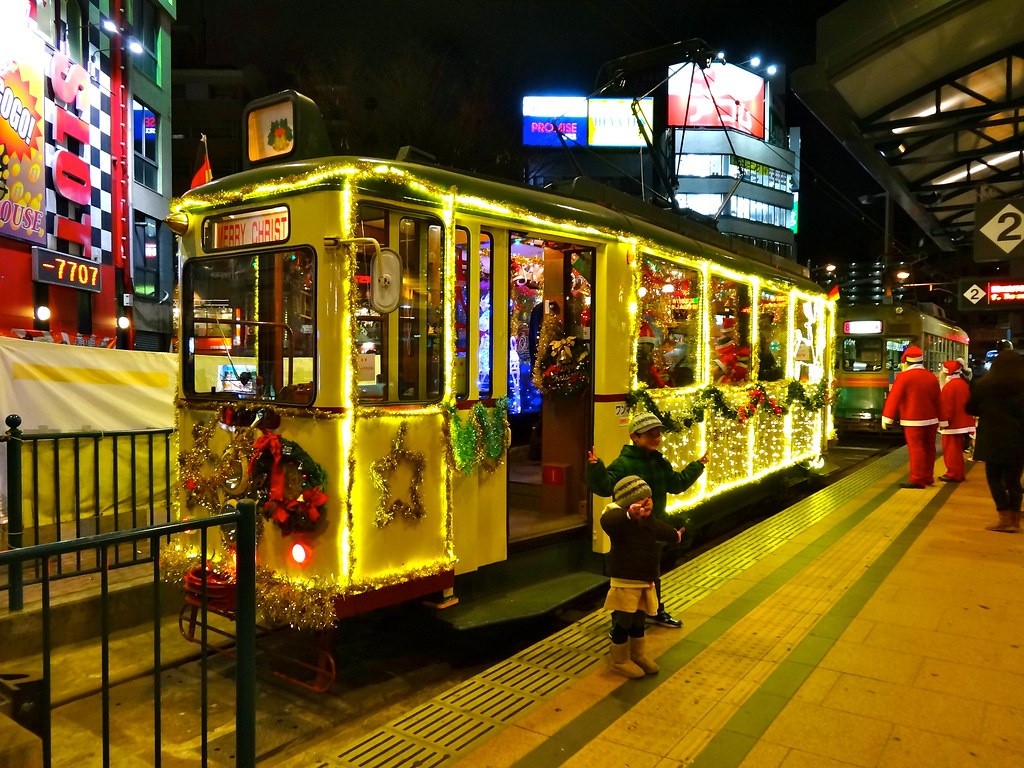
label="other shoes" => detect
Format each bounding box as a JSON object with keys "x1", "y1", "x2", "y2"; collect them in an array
[
  {"x1": 898, "y1": 482, "x2": 934, "y2": 489},
  {"x1": 938, "y1": 474, "x2": 966, "y2": 482}
]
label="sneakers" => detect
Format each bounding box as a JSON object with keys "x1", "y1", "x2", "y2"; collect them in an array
[{"x1": 645, "y1": 610, "x2": 682, "y2": 627}]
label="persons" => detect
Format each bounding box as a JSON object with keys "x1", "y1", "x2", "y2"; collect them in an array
[
  {"x1": 600, "y1": 475, "x2": 685, "y2": 680},
  {"x1": 527, "y1": 301, "x2": 560, "y2": 464},
  {"x1": 588, "y1": 414, "x2": 708, "y2": 629},
  {"x1": 881, "y1": 339, "x2": 1024, "y2": 533}
]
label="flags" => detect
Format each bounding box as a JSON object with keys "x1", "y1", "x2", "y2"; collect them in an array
[{"x1": 190, "y1": 155, "x2": 213, "y2": 192}]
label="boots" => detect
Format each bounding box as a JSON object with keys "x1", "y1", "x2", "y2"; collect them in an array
[
  {"x1": 609, "y1": 637, "x2": 660, "y2": 678},
  {"x1": 985, "y1": 511, "x2": 1021, "y2": 532}
]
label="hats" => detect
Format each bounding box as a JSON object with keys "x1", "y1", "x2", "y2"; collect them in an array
[
  {"x1": 613, "y1": 475, "x2": 652, "y2": 509},
  {"x1": 901, "y1": 346, "x2": 923, "y2": 366},
  {"x1": 628, "y1": 412, "x2": 665, "y2": 435},
  {"x1": 943, "y1": 360, "x2": 961, "y2": 382}
]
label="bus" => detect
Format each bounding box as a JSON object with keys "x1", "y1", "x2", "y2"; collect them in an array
[
  {"x1": 164, "y1": 90, "x2": 838, "y2": 691},
  {"x1": 837, "y1": 296, "x2": 970, "y2": 433}
]
